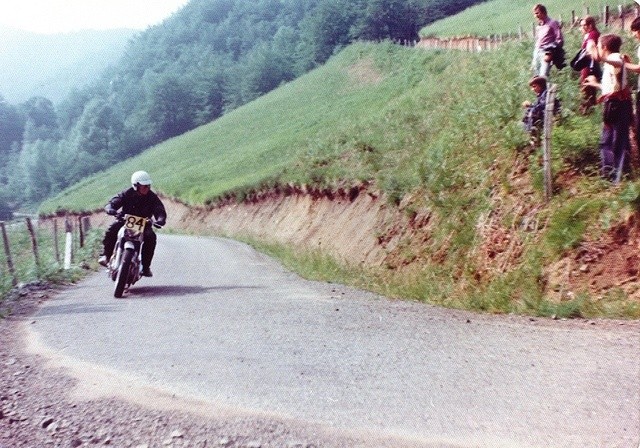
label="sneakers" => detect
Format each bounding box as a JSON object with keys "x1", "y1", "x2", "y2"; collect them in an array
[
  {"x1": 142, "y1": 268, "x2": 152, "y2": 277},
  {"x1": 98, "y1": 254, "x2": 108, "y2": 266}
]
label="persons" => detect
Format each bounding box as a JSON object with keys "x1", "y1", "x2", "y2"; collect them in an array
[
  {"x1": 622, "y1": 16, "x2": 640, "y2": 80},
  {"x1": 521, "y1": 75, "x2": 560, "y2": 146},
  {"x1": 572, "y1": 16, "x2": 604, "y2": 114},
  {"x1": 583, "y1": 34, "x2": 634, "y2": 180},
  {"x1": 98, "y1": 171, "x2": 166, "y2": 276},
  {"x1": 531, "y1": 4, "x2": 564, "y2": 88}
]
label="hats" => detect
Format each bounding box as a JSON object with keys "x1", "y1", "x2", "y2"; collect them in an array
[{"x1": 529, "y1": 75, "x2": 550, "y2": 85}]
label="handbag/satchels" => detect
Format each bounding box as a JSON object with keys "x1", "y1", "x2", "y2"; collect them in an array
[{"x1": 604, "y1": 98, "x2": 632, "y2": 125}]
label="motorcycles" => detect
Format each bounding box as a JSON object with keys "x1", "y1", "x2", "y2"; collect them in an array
[{"x1": 99, "y1": 212, "x2": 161, "y2": 298}]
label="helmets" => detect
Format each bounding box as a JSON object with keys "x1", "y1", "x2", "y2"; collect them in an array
[{"x1": 131, "y1": 171, "x2": 152, "y2": 191}]
